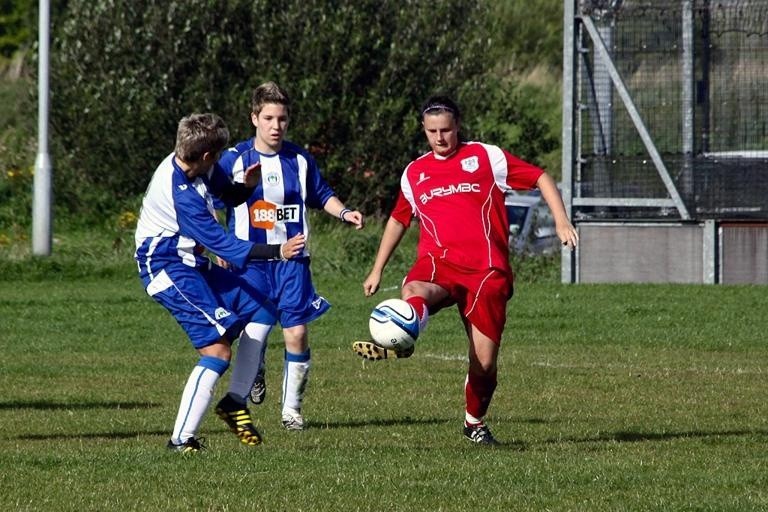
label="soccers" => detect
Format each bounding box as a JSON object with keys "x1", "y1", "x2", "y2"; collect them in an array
[{"x1": 369, "y1": 298, "x2": 420, "y2": 350}]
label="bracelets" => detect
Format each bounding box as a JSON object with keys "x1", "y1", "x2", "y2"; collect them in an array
[{"x1": 341, "y1": 208, "x2": 351, "y2": 221}]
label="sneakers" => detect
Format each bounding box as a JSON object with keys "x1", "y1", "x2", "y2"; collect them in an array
[
  {"x1": 250, "y1": 359, "x2": 266, "y2": 405},
  {"x1": 213, "y1": 393, "x2": 262, "y2": 446},
  {"x1": 462, "y1": 419, "x2": 496, "y2": 445},
  {"x1": 352, "y1": 340, "x2": 415, "y2": 360},
  {"x1": 167, "y1": 437, "x2": 206, "y2": 453},
  {"x1": 282, "y1": 413, "x2": 304, "y2": 429}
]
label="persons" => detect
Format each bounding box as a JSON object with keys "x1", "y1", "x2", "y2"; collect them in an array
[
  {"x1": 351, "y1": 96, "x2": 579, "y2": 448},
  {"x1": 212, "y1": 81, "x2": 364, "y2": 433},
  {"x1": 134, "y1": 113, "x2": 306, "y2": 452}
]
